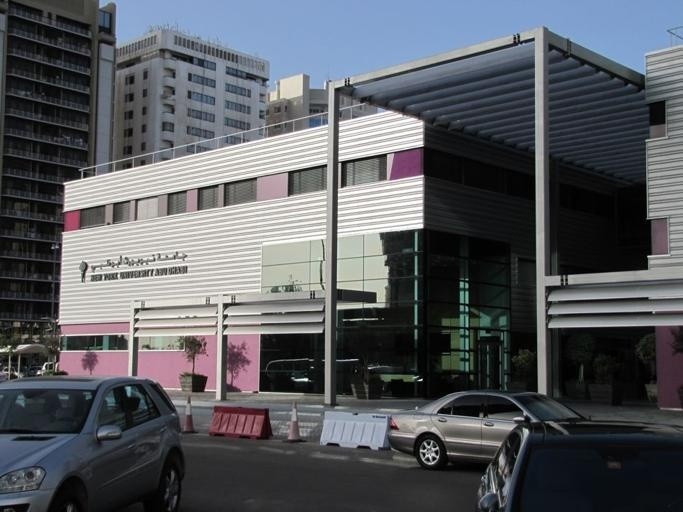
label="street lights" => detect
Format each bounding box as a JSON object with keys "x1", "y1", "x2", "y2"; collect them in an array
[{"x1": 49, "y1": 240, "x2": 60, "y2": 320}]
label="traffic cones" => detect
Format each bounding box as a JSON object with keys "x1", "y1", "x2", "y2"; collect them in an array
[
  {"x1": 181, "y1": 395, "x2": 199, "y2": 433},
  {"x1": 282, "y1": 399, "x2": 308, "y2": 444}
]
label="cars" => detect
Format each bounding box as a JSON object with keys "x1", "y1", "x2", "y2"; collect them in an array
[
  {"x1": 385, "y1": 386, "x2": 588, "y2": 473},
  {"x1": 266, "y1": 357, "x2": 475, "y2": 399},
  {"x1": 0, "y1": 374, "x2": 190, "y2": 511},
  {"x1": 0, "y1": 361, "x2": 54, "y2": 379}
]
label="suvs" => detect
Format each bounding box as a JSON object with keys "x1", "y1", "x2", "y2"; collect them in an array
[{"x1": 471, "y1": 412, "x2": 683, "y2": 510}]
